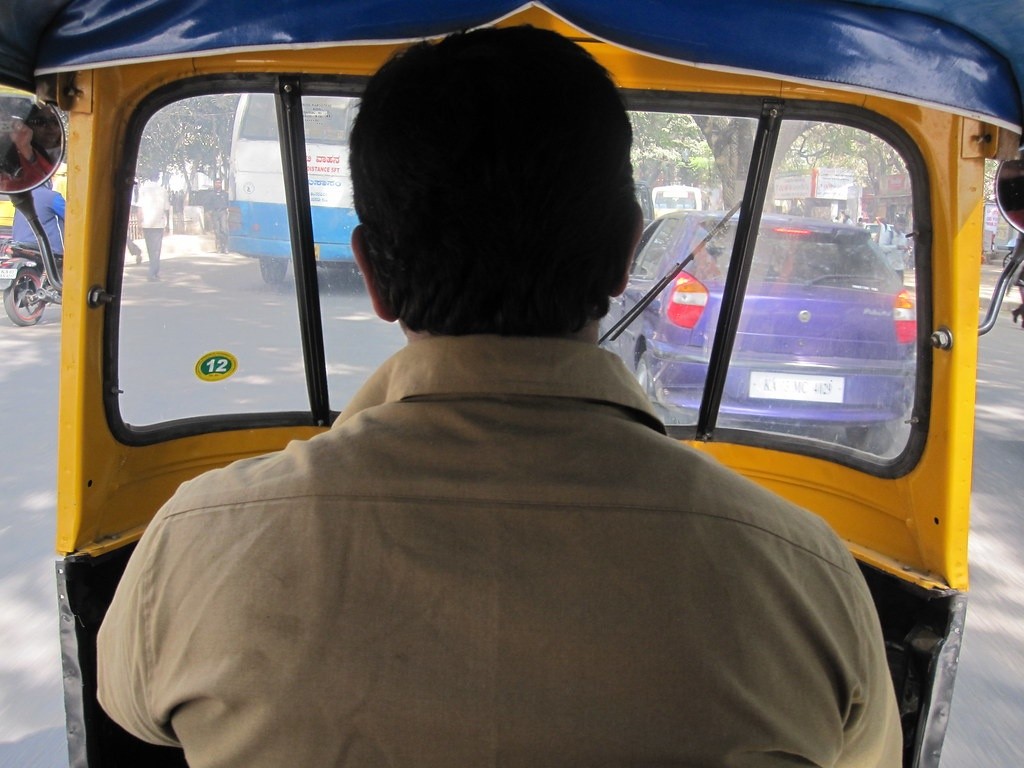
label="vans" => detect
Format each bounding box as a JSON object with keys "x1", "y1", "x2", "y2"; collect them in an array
[{"x1": 634, "y1": 181, "x2": 655, "y2": 237}]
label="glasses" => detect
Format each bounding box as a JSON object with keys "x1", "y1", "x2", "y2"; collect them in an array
[{"x1": 25, "y1": 115, "x2": 61, "y2": 128}]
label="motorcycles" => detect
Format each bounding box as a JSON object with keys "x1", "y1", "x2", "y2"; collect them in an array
[{"x1": 2, "y1": 240, "x2": 62, "y2": 327}]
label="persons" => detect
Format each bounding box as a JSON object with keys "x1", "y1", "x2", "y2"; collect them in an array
[
  {"x1": 135, "y1": 169, "x2": 170, "y2": 281},
  {"x1": 126, "y1": 237, "x2": 142, "y2": 264},
  {"x1": 97, "y1": 25, "x2": 902, "y2": 768},
  {"x1": 208, "y1": 178, "x2": 229, "y2": 254},
  {"x1": 834, "y1": 210, "x2": 863, "y2": 228},
  {"x1": 880, "y1": 217, "x2": 912, "y2": 286},
  {"x1": 1012, "y1": 286, "x2": 1024, "y2": 328},
  {"x1": 0, "y1": 107, "x2": 60, "y2": 191},
  {"x1": 876, "y1": 216, "x2": 891, "y2": 248},
  {"x1": 12, "y1": 177, "x2": 66, "y2": 255}
]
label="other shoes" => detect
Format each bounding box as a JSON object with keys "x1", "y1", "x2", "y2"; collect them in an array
[{"x1": 146, "y1": 273, "x2": 162, "y2": 282}]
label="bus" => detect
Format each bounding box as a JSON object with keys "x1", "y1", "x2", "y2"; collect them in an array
[
  {"x1": 652, "y1": 185, "x2": 711, "y2": 221},
  {"x1": 227, "y1": 93, "x2": 362, "y2": 287}
]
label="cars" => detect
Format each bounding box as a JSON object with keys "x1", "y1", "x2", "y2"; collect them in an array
[
  {"x1": 600, "y1": 210, "x2": 917, "y2": 457},
  {"x1": 861, "y1": 221, "x2": 895, "y2": 243}
]
